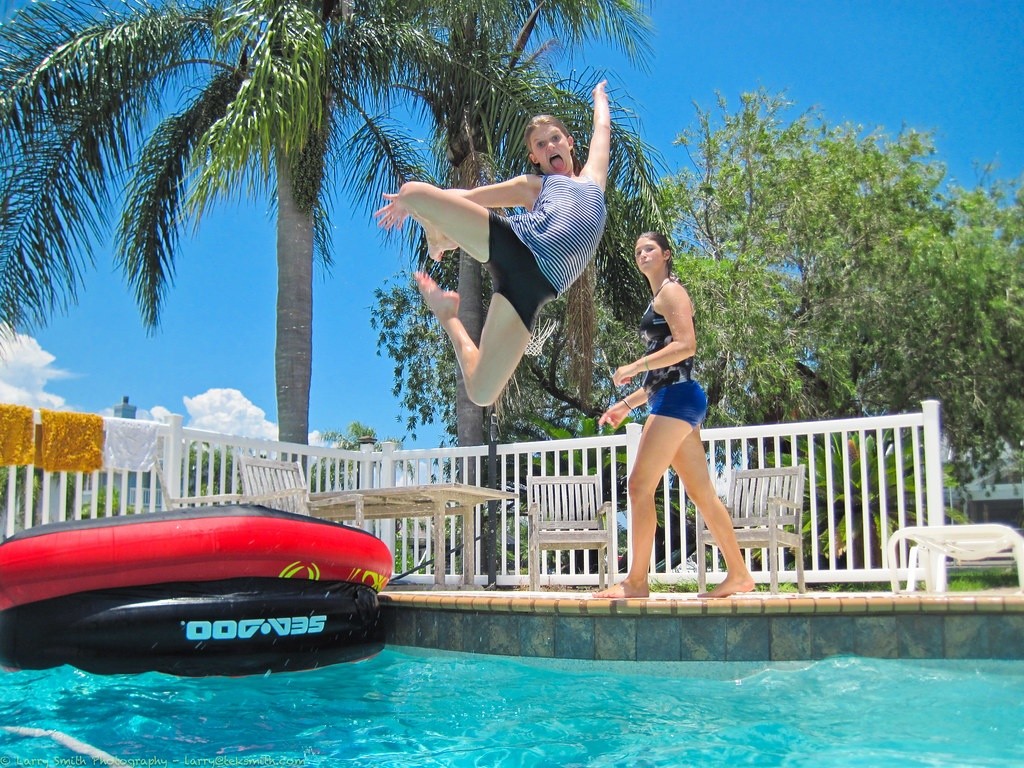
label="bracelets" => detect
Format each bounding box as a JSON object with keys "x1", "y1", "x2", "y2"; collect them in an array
[
  {"x1": 622, "y1": 399, "x2": 632, "y2": 410},
  {"x1": 645, "y1": 356, "x2": 649, "y2": 370}
]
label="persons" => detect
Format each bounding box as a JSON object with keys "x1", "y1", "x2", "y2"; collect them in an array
[
  {"x1": 374, "y1": 80, "x2": 610, "y2": 406},
  {"x1": 592, "y1": 232, "x2": 755, "y2": 598}
]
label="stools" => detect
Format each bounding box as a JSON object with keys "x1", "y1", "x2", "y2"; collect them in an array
[{"x1": 888, "y1": 523, "x2": 1024, "y2": 597}]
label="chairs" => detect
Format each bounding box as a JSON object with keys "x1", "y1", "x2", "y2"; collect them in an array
[
  {"x1": 526, "y1": 474, "x2": 614, "y2": 592},
  {"x1": 238, "y1": 453, "x2": 364, "y2": 529},
  {"x1": 152, "y1": 455, "x2": 245, "y2": 511},
  {"x1": 697, "y1": 464, "x2": 807, "y2": 596}
]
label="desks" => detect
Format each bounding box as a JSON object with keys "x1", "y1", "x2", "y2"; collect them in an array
[{"x1": 307, "y1": 483, "x2": 518, "y2": 590}]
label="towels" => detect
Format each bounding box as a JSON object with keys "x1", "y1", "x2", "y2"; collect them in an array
[
  {"x1": 105, "y1": 416, "x2": 159, "y2": 472},
  {"x1": 0, "y1": 402, "x2": 37, "y2": 469},
  {"x1": 33, "y1": 407, "x2": 104, "y2": 472}
]
